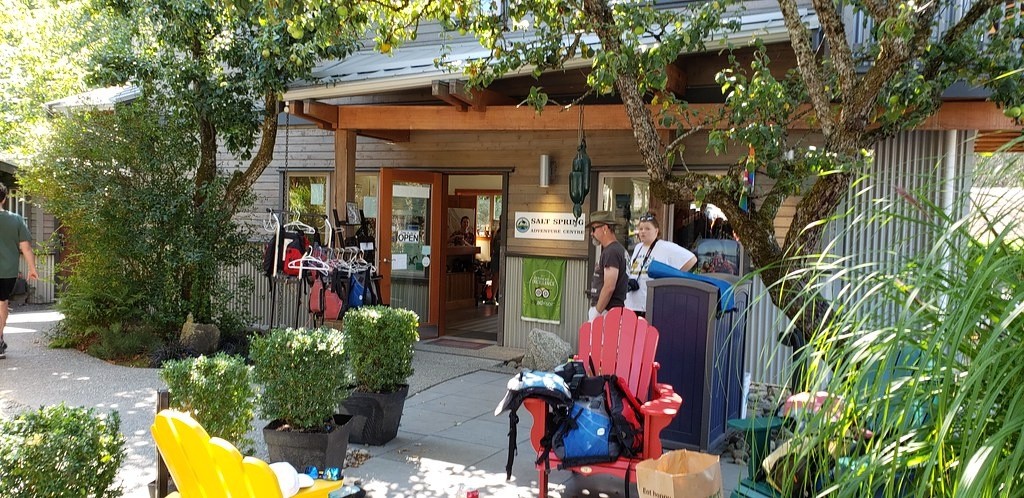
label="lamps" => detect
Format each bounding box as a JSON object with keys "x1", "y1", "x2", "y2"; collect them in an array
[{"x1": 540, "y1": 153, "x2": 551, "y2": 187}]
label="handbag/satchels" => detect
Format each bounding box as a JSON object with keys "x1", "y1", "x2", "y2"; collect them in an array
[{"x1": 636, "y1": 449, "x2": 725, "y2": 498}]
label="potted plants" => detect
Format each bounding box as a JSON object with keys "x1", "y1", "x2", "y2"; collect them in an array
[
  {"x1": 147, "y1": 351, "x2": 260, "y2": 498},
  {"x1": 247, "y1": 326, "x2": 352, "y2": 477},
  {"x1": 336, "y1": 305, "x2": 420, "y2": 444}
]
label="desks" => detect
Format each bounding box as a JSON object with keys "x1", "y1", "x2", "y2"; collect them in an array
[{"x1": 445, "y1": 246, "x2": 481, "y2": 310}]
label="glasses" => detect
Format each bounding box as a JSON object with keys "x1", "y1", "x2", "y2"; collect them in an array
[
  {"x1": 305, "y1": 466, "x2": 343, "y2": 481},
  {"x1": 641, "y1": 216, "x2": 654, "y2": 222},
  {"x1": 591, "y1": 223, "x2": 606, "y2": 233}
]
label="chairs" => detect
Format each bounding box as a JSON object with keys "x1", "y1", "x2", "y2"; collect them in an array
[
  {"x1": 522, "y1": 307, "x2": 683, "y2": 498},
  {"x1": 150, "y1": 410, "x2": 344, "y2": 498},
  {"x1": 726, "y1": 346, "x2": 946, "y2": 498}
]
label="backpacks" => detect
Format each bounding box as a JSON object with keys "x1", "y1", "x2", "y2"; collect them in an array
[{"x1": 494, "y1": 361, "x2": 644, "y2": 481}]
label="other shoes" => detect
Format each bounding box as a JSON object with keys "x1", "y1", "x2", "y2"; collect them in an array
[
  {"x1": 489, "y1": 296, "x2": 498, "y2": 306},
  {"x1": 0, "y1": 340, "x2": 6, "y2": 359}
]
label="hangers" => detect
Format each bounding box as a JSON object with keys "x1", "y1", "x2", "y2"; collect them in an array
[{"x1": 262, "y1": 207, "x2": 377, "y2": 274}]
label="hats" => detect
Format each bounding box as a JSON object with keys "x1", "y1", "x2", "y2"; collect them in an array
[{"x1": 586, "y1": 211, "x2": 622, "y2": 227}]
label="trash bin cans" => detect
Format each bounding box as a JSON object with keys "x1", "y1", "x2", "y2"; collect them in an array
[
  {"x1": 19, "y1": 252, "x2": 55, "y2": 304},
  {"x1": 643, "y1": 272, "x2": 753, "y2": 453}
]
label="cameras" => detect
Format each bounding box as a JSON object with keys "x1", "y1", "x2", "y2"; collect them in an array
[{"x1": 628, "y1": 278, "x2": 639, "y2": 292}]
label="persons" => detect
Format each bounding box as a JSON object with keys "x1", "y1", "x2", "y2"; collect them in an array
[
  {"x1": 446, "y1": 216, "x2": 475, "y2": 247},
  {"x1": 491, "y1": 215, "x2": 501, "y2": 305},
  {"x1": 624, "y1": 208, "x2": 698, "y2": 318},
  {"x1": 0, "y1": 182, "x2": 39, "y2": 359},
  {"x1": 583, "y1": 209, "x2": 630, "y2": 360}
]
